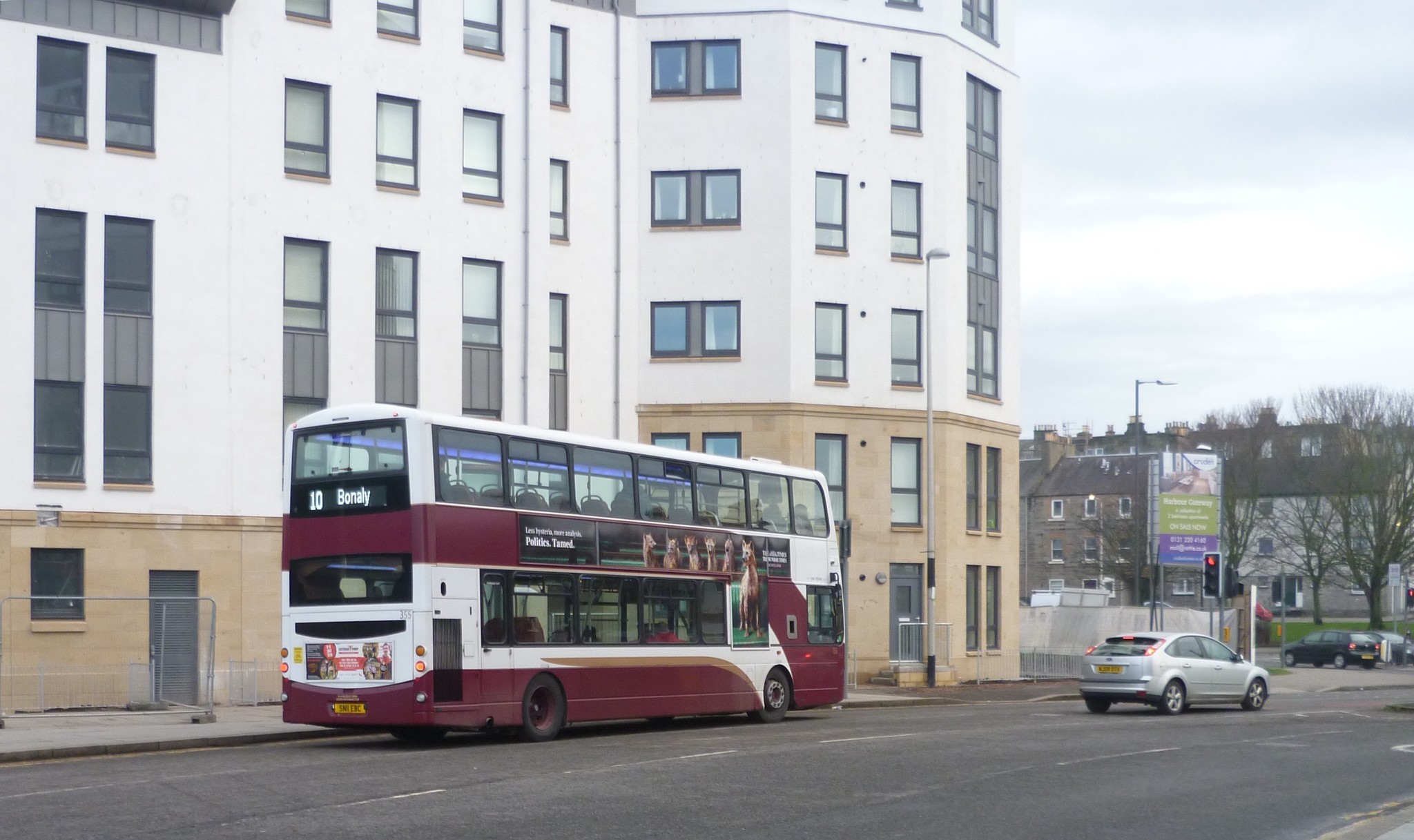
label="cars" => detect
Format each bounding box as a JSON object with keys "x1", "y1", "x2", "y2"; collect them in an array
[
  {"x1": 1143, "y1": 601, "x2": 1174, "y2": 607},
  {"x1": 1077, "y1": 630, "x2": 1272, "y2": 717},
  {"x1": 1364, "y1": 631, "x2": 1414, "y2": 665},
  {"x1": 1279, "y1": 629, "x2": 1380, "y2": 670}
]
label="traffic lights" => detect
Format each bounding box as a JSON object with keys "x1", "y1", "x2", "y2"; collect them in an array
[
  {"x1": 1203, "y1": 552, "x2": 1222, "y2": 598},
  {"x1": 1406, "y1": 588, "x2": 1414, "y2": 607}
]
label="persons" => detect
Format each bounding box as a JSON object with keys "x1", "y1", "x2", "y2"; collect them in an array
[
  {"x1": 645, "y1": 621, "x2": 678, "y2": 642},
  {"x1": 378, "y1": 644, "x2": 392, "y2": 665},
  {"x1": 563, "y1": 625, "x2": 599, "y2": 644},
  {"x1": 615, "y1": 481, "x2": 654, "y2": 520}
]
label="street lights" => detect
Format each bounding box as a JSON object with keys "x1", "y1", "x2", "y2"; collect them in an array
[
  {"x1": 1089, "y1": 495, "x2": 1102, "y2": 591},
  {"x1": 1135, "y1": 381, "x2": 1177, "y2": 631},
  {"x1": 925, "y1": 246, "x2": 951, "y2": 686}
]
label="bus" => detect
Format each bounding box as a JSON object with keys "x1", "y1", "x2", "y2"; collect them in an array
[{"x1": 280, "y1": 403, "x2": 846, "y2": 742}]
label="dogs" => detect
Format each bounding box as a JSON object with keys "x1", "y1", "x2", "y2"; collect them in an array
[
  {"x1": 739, "y1": 539, "x2": 762, "y2": 638},
  {"x1": 644, "y1": 528, "x2": 735, "y2": 572}
]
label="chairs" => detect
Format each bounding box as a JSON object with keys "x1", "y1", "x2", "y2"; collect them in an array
[
  {"x1": 450, "y1": 479, "x2": 720, "y2": 527},
  {"x1": 485, "y1": 617, "x2": 602, "y2": 644}
]
